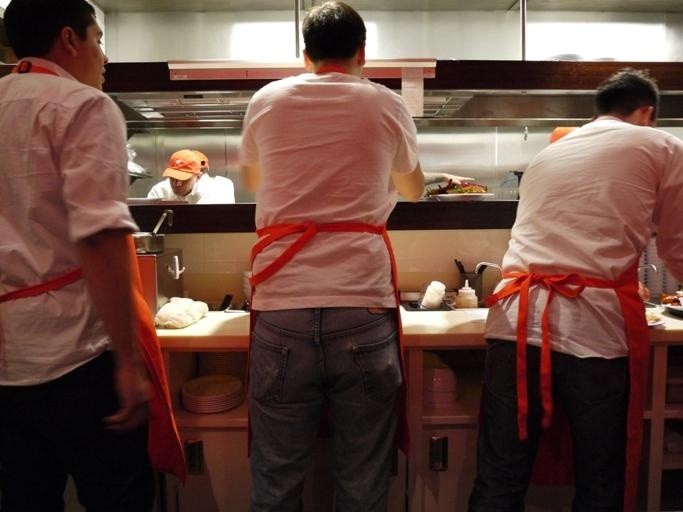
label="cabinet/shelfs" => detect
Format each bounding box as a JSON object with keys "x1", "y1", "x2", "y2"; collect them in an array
[
  {"x1": 399, "y1": 331, "x2": 579, "y2": 510},
  {"x1": 642, "y1": 331, "x2": 683, "y2": 512},
  {"x1": 153, "y1": 334, "x2": 407, "y2": 512}
]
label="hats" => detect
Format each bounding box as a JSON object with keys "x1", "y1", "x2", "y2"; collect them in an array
[
  {"x1": 162, "y1": 149, "x2": 201, "y2": 180},
  {"x1": 550, "y1": 127, "x2": 579, "y2": 143},
  {"x1": 192, "y1": 150, "x2": 209, "y2": 171}
]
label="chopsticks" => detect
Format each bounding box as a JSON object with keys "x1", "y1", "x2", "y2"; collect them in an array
[{"x1": 454, "y1": 257, "x2": 487, "y2": 276}]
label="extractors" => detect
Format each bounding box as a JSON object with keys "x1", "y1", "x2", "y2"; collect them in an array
[{"x1": 0, "y1": 0, "x2": 683, "y2": 128}]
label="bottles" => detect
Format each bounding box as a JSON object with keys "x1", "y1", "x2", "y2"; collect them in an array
[
  {"x1": 456, "y1": 278, "x2": 478, "y2": 308},
  {"x1": 419, "y1": 281, "x2": 446, "y2": 310}
]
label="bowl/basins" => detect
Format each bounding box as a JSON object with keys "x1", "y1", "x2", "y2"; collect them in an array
[
  {"x1": 132, "y1": 208, "x2": 172, "y2": 253},
  {"x1": 421, "y1": 353, "x2": 457, "y2": 412},
  {"x1": 638, "y1": 236, "x2": 678, "y2": 298},
  {"x1": 241, "y1": 270, "x2": 251, "y2": 303}
]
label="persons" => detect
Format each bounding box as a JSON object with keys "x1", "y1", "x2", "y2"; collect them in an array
[
  {"x1": 192, "y1": 150, "x2": 236, "y2": 206},
  {"x1": 1, "y1": 1, "x2": 148, "y2": 512},
  {"x1": 147, "y1": 150, "x2": 217, "y2": 204},
  {"x1": 466, "y1": 66, "x2": 683, "y2": 511},
  {"x1": 238, "y1": 0, "x2": 426, "y2": 511}
]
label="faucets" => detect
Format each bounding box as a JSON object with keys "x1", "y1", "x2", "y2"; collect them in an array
[{"x1": 635, "y1": 262, "x2": 658, "y2": 275}]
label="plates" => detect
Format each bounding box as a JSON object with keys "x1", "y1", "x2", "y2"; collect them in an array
[
  {"x1": 643, "y1": 290, "x2": 682, "y2": 328},
  {"x1": 180, "y1": 373, "x2": 247, "y2": 413},
  {"x1": 428, "y1": 193, "x2": 495, "y2": 201}
]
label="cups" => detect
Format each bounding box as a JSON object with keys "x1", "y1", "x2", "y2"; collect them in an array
[
  {"x1": 457, "y1": 271, "x2": 482, "y2": 299},
  {"x1": 429, "y1": 434, "x2": 448, "y2": 471},
  {"x1": 184, "y1": 439, "x2": 204, "y2": 474}
]
